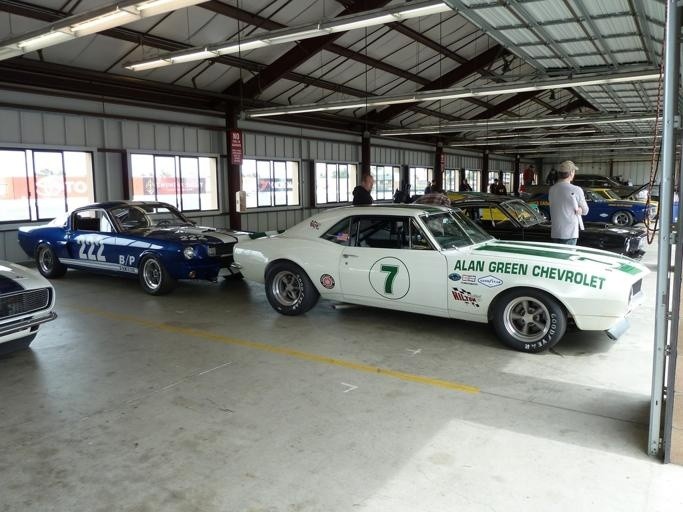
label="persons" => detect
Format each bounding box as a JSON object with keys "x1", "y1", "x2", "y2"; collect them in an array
[
  {"x1": 425, "y1": 182, "x2": 432, "y2": 194},
  {"x1": 523, "y1": 164, "x2": 537, "y2": 188},
  {"x1": 546, "y1": 165, "x2": 558, "y2": 186},
  {"x1": 490, "y1": 179, "x2": 500, "y2": 195},
  {"x1": 352, "y1": 174, "x2": 374, "y2": 204},
  {"x1": 431, "y1": 180, "x2": 443, "y2": 193},
  {"x1": 547, "y1": 159, "x2": 589, "y2": 246},
  {"x1": 460, "y1": 178, "x2": 472, "y2": 192},
  {"x1": 497, "y1": 182, "x2": 506, "y2": 195}
]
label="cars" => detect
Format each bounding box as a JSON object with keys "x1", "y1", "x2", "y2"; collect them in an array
[
  {"x1": 0, "y1": 259, "x2": 57, "y2": 354},
  {"x1": 581, "y1": 188, "x2": 658, "y2": 218},
  {"x1": 443, "y1": 189, "x2": 539, "y2": 222},
  {"x1": 17, "y1": 199, "x2": 257, "y2": 296},
  {"x1": 451, "y1": 198, "x2": 647, "y2": 261},
  {"x1": 234, "y1": 203, "x2": 650, "y2": 354},
  {"x1": 529, "y1": 187, "x2": 655, "y2": 227}
]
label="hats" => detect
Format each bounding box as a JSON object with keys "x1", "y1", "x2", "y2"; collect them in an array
[{"x1": 557, "y1": 161, "x2": 579, "y2": 172}]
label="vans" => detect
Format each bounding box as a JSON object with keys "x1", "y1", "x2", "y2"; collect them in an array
[{"x1": 571, "y1": 173, "x2": 648, "y2": 202}]
